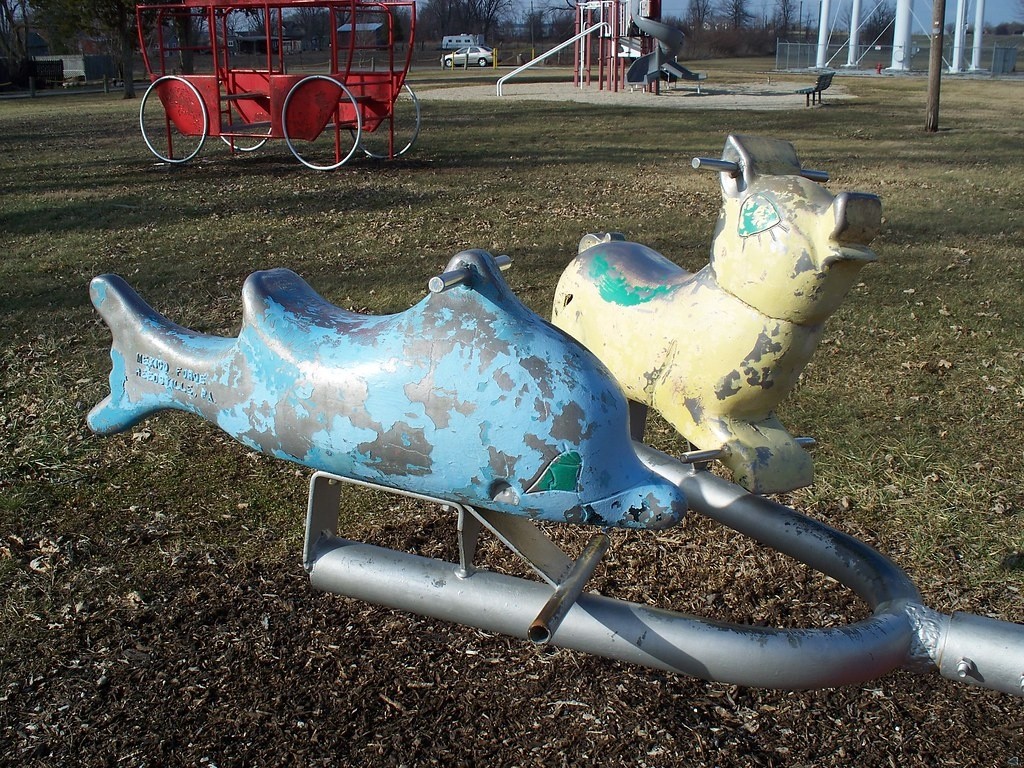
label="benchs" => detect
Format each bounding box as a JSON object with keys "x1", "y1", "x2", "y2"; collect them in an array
[
  {"x1": 794, "y1": 72, "x2": 836, "y2": 107},
  {"x1": 340, "y1": 95, "x2": 371, "y2": 104},
  {"x1": 220, "y1": 92, "x2": 263, "y2": 101}
]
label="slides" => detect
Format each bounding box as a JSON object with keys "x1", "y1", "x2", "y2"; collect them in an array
[{"x1": 624, "y1": 14, "x2": 708, "y2": 85}]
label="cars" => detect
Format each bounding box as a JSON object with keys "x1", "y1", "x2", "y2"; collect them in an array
[{"x1": 439, "y1": 45, "x2": 500, "y2": 67}]
